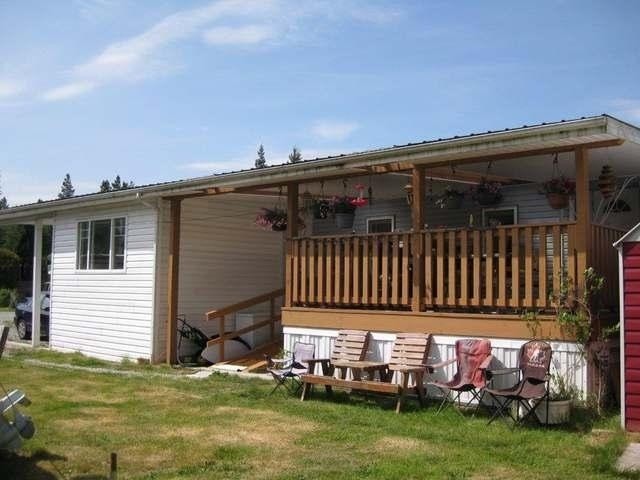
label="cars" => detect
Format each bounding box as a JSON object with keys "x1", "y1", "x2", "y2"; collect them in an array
[{"x1": 13, "y1": 291, "x2": 49, "y2": 339}]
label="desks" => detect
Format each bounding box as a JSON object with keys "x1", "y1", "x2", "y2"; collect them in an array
[{"x1": 333, "y1": 359, "x2": 384, "y2": 384}]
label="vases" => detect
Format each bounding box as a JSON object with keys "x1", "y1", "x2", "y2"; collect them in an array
[{"x1": 550, "y1": 194, "x2": 572, "y2": 209}]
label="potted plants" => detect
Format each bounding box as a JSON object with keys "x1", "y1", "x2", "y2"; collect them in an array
[{"x1": 531, "y1": 373, "x2": 575, "y2": 424}]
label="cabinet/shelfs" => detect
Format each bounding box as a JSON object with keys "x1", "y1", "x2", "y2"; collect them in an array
[{"x1": 235, "y1": 311, "x2": 281, "y2": 348}]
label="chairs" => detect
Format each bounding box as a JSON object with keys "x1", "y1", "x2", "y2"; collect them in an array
[
  {"x1": 365, "y1": 333, "x2": 435, "y2": 422},
  {"x1": 482, "y1": 339, "x2": 557, "y2": 431},
  {"x1": 265, "y1": 341, "x2": 316, "y2": 398},
  {"x1": 428, "y1": 337, "x2": 494, "y2": 424},
  {"x1": 301, "y1": 329, "x2": 373, "y2": 401}
]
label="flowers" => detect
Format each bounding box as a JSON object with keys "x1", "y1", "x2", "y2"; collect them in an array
[
  {"x1": 536, "y1": 177, "x2": 577, "y2": 196},
  {"x1": 256, "y1": 159, "x2": 505, "y2": 235}
]
